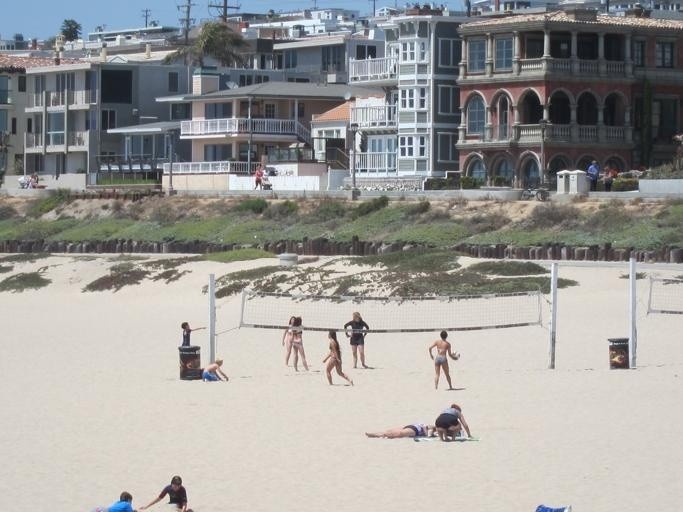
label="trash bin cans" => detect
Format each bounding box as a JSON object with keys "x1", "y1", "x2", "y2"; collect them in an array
[
  {"x1": 179, "y1": 345, "x2": 202, "y2": 380},
  {"x1": 608, "y1": 338, "x2": 629, "y2": 369}
]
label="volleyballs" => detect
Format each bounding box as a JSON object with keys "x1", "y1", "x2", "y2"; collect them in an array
[{"x1": 452, "y1": 350, "x2": 459, "y2": 359}]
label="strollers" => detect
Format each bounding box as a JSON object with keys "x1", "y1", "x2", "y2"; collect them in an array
[{"x1": 259, "y1": 176, "x2": 272, "y2": 190}]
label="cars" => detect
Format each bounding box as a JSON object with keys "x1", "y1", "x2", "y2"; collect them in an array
[{"x1": 263, "y1": 167, "x2": 278, "y2": 176}]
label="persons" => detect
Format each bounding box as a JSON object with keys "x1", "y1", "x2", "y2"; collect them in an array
[
  {"x1": 364, "y1": 422, "x2": 462, "y2": 438},
  {"x1": 254, "y1": 164, "x2": 262, "y2": 191},
  {"x1": 138, "y1": 474, "x2": 187, "y2": 511},
  {"x1": 90, "y1": 490, "x2": 138, "y2": 512},
  {"x1": 586, "y1": 160, "x2": 600, "y2": 192},
  {"x1": 605, "y1": 167, "x2": 618, "y2": 192},
  {"x1": 199, "y1": 357, "x2": 229, "y2": 383},
  {"x1": 342, "y1": 311, "x2": 369, "y2": 369},
  {"x1": 426, "y1": 330, "x2": 460, "y2": 389},
  {"x1": 321, "y1": 331, "x2": 354, "y2": 386},
  {"x1": 281, "y1": 315, "x2": 296, "y2": 366},
  {"x1": 25, "y1": 172, "x2": 38, "y2": 189},
  {"x1": 433, "y1": 403, "x2": 472, "y2": 442},
  {"x1": 289, "y1": 317, "x2": 311, "y2": 372},
  {"x1": 180, "y1": 321, "x2": 207, "y2": 346}
]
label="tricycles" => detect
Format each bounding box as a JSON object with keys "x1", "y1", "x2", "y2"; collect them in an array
[{"x1": 20, "y1": 177, "x2": 48, "y2": 190}]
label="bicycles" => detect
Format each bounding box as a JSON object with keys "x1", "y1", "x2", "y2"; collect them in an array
[{"x1": 522, "y1": 188, "x2": 546, "y2": 201}]
